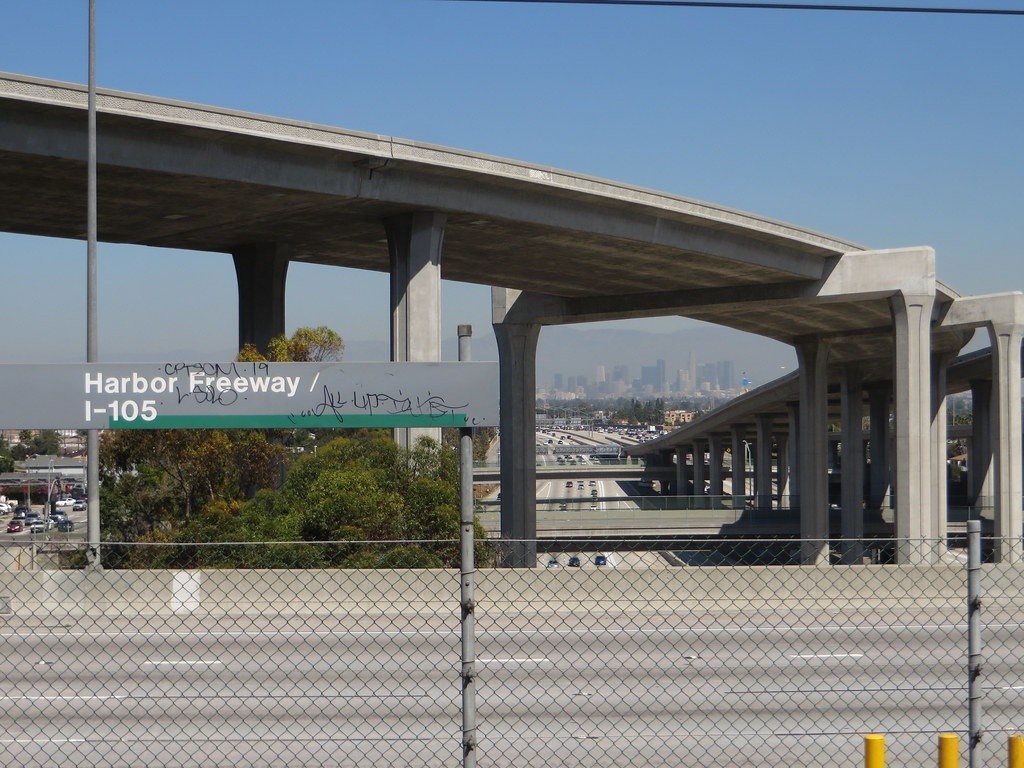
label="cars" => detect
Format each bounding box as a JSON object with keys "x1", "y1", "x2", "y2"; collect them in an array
[
  {"x1": 547, "y1": 560, "x2": 559, "y2": 568},
  {"x1": 595, "y1": 555, "x2": 607, "y2": 565},
  {"x1": 569, "y1": 557, "x2": 580, "y2": 567},
  {"x1": 537, "y1": 422, "x2": 676, "y2": 510},
  {"x1": 0, "y1": 484, "x2": 88, "y2": 533}
]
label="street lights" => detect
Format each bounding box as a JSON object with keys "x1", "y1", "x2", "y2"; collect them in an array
[{"x1": 26, "y1": 454, "x2": 40, "y2": 509}]
